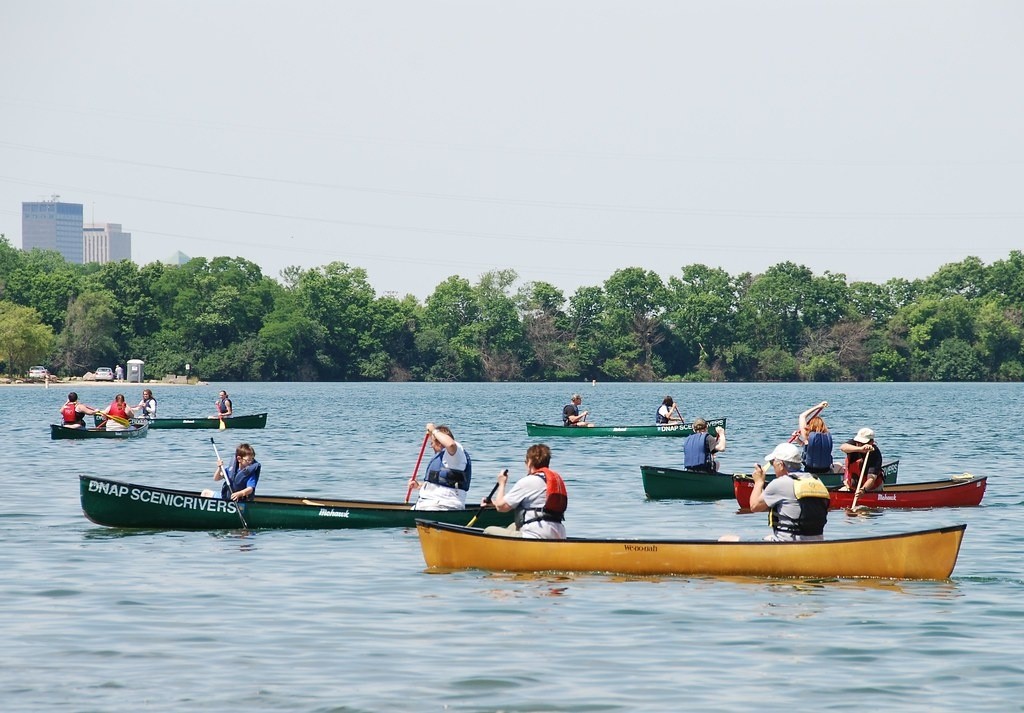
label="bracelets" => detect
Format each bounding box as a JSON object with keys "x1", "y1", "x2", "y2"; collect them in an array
[{"x1": 432, "y1": 428, "x2": 440, "y2": 436}]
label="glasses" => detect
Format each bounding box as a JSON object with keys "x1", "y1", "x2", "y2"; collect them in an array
[
  {"x1": 770, "y1": 458, "x2": 776, "y2": 465},
  {"x1": 242, "y1": 457, "x2": 255, "y2": 465}
]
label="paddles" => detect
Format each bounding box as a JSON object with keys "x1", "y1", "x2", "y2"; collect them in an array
[
  {"x1": 466, "y1": 469, "x2": 509, "y2": 527},
  {"x1": 210, "y1": 437, "x2": 248, "y2": 530},
  {"x1": 584, "y1": 415, "x2": 586, "y2": 422},
  {"x1": 675, "y1": 406, "x2": 684, "y2": 424},
  {"x1": 96, "y1": 421, "x2": 106, "y2": 429},
  {"x1": 130, "y1": 405, "x2": 143, "y2": 408},
  {"x1": 763, "y1": 403, "x2": 827, "y2": 471},
  {"x1": 851, "y1": 448, "x2": 874, "y2": 508},
  {"x1": 84, "y1": 405, "x2": 129, "y2": 428},
  {"x1": 405, "y1": 429, "x2": 431, "y2": 502},
  {"x1": 218, "y1": 403, "x2": 225, "y2": 430},
  {"x1": 712, "y1": 433, "x2": 720, "y2": 459}
]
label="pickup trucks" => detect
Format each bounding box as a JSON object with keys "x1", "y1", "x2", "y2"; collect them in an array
[{"x1": 27, "y1": 366, "x2": 47, "y2": 380}]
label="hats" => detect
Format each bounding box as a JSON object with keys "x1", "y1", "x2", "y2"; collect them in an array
[
  {"x1": 854, "y1": 428, "x2": 875, "y2": 443},
  {"x1": 571, "y1": 394, "x2": 582, "y2": 401},
  {"x1": 764, "y1": 442, "x2": 803, "y2": 463}
]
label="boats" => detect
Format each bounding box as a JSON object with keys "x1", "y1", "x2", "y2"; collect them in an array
[
  {"x1": 639, "y1": 459, "x2": 901, "y2": 501},
  {"x1": 93, "y1": 411, "x2": 268, "y2": 430},
  {"x1": 49, "y1": 421, "x2": 149, "y2": 441},
  {"x1": 526, "y1": 417, "x2": 727, "y2": 438},
  {"x1": 78, "y1": 473, "x2": 516, "y2": 531},
  {"x1": 731, "y1": 472, "x2": 989, "y2": 511},
  {"x1": 414, "y1": 516, "x2": 968, "y2": 580}
]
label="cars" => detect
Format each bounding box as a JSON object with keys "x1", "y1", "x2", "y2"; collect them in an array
[{"x1": 94, "y1": 367, "x2": 114, "y2": 382}]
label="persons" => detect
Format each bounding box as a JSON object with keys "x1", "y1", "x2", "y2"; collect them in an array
[
  {"x1": 408, "y1": 423, "x2": 472, "y2": 511},
  {"x1": 684, "y1": 418, "x2": 726, "y2": 472},
  {"x1": 480, "y1": 444, "x2": 567, "y2": 539},
  {"x1": 656, "y1": 396, "x2": 684, "y2": 425},
  {"x1": 750, "y1": 442, "x2": 830, "y2": 542},
  {"x1": 115, "y1": 364, "x2": 125, "y2": 382},
  {"x1": 207, "y1": 390, "x2": 233, "y2": 419},
  {"x1": 562, "y1": 394, "x2": 595, "y2": 427},
  {"x1": 791, "y1": 401, "x2": 835, "y2": 475},
  {"x1": 60, "y1": 392, "x2": 100, "y2": 431},
  {"x1": 200, "y1": 444, "x2": 261, "y2": 503},
  {"x1": 102, "y1": 393, "x2": 138, "y2": 431},
  {"x1": 839, "y1": 428, "x2": 884, "y2": 497},
  {"x1": 131, "y1": 389, "x2": 157, "y2": 419}
]
